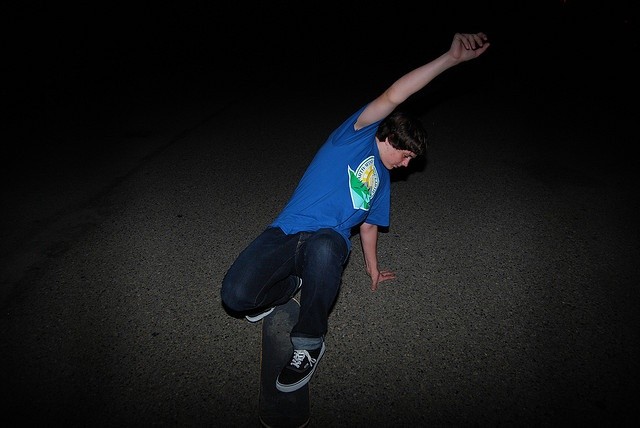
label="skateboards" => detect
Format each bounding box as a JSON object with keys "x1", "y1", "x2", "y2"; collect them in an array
[{"x1": 258, "y1": 298, "x2": 311, "y2": 428}]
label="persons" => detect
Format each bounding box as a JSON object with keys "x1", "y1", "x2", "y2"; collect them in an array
[{"x1": 220, "y1": 32, "x2": 490, "y2": 393}]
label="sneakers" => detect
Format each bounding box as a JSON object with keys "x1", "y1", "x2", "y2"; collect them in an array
[
  {"x1": 245, "y1": 278, "x2": 302, "y2": 322},
  {"x1": 276, "y1": 342, "x2": 326, "y2": 392}
]
